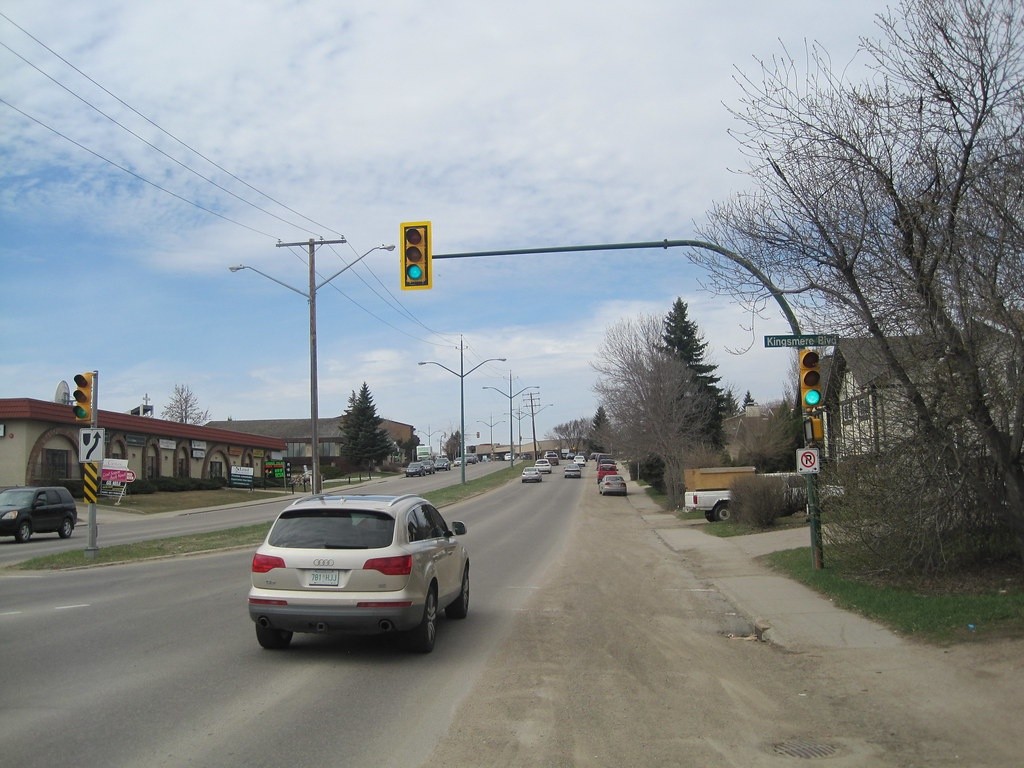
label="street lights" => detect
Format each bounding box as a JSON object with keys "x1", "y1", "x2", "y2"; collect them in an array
[
  {"x1": 481, "y1": 385, "x2": 539, "y2": 466},
  {"x1": 227, "y1": 244, "x2": 396, "y2": 494},
  {"x1": 418, "y1": 430, "x2": 441, "y2": 444},
  {"x1": 504, "y1": 403, "x2": 553, "y2": 459},
  {"x1": 419, "y1": 359, "x2": 507, "y2": 482},
  {"x1": 476, "y1": 420, "x2": 506, "y2": 459}
]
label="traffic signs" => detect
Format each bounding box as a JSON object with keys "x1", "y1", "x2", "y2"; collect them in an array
[{"x1": 764, "y1": 336, "x2": 838, "y2": 347}]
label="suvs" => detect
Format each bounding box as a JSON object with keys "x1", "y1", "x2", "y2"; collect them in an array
[
  {"x1": 0, "y1": 486, "x2": 80, "y2": 542},
  {"x1": 248, "y1": 495, "x2": 471, "y2": 651},
  {"x1": 434, "y1": 458, "x2": 451, "y2": 471},
  {"x1": 421, "y1": 460, "x2": 435, "y2": 474},
  {"x1": 406, "y1": 462, "x2": 427, "y2": 476}
]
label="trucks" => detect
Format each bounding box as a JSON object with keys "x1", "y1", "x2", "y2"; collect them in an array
[{"x1": 416, "y1": 445, "x2": 432, "y2": 461}]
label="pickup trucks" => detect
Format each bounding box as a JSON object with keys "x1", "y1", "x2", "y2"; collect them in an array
[{"x1": 683, "y1": 474, "x2": 849, "y2": 522}]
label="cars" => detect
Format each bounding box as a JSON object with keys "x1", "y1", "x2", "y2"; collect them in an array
[
  {"x1": 599, "y1": 475, "x2": 627, "y2": 496},
  {"x1": 465, "y1": 453, "x2": 478, "y2": 464},
  {"x1": 454, "y1": 457, "x2": 467, "y2": 466},
  {"x1": 504, "y1": 453, "x2": 515, "y2": 461},
  {"x1": 521, "y1": 467, "x2": 542, "y2": 483},
  {"x1": 517, "y1": 453, "x2": 530, "y2": 460},
  {"x1": 544, "y1": 451, "x2": 559, "y2": 465},
  {"x1": 535, "y1": 459, "x2": 552, "y2": 474},
  {"x1": 561, "y1": 449, "x2": 619, "y2": 483}
]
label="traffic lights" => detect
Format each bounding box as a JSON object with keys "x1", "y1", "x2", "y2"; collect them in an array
[
  {"x1": 799, "y1": 349, "x2": 822, "y2": 407},
  {"x1": 73, "y1": 372, "x2": 92, "y2": 423},
  {"x1": 400, "y1": 222, "x2": 433, "y2": 289},
  {"x1": 803, "y1": 416, "x2": 824, "y2": 441}
]
